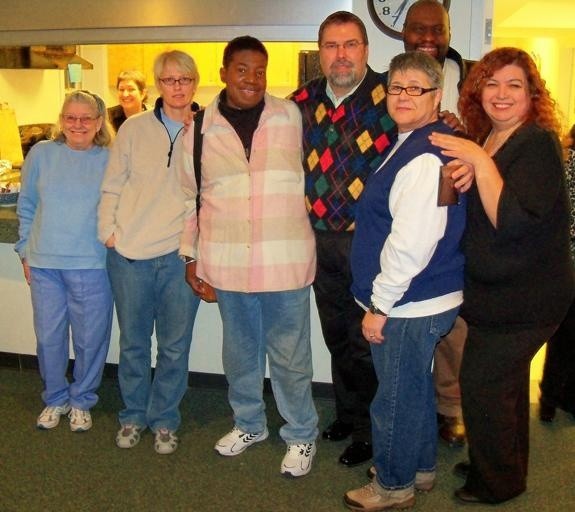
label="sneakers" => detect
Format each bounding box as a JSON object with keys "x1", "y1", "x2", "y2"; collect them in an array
[
  {"x1": 37, "y1": 404, "x2": 71, "y2": 429},
  {"x1": 368, "y1": 465, "x2": 439, "y2": 492},
  {"x1": 280, "y1": 444, "x2": 317, "y2": 478},
  {"x1": 115, "y1": 424, "x2": 146, "y2": 447},
  {"x1": 340, "y1": 479, "x2": 418, "y2": 511},
  {"x1": 437, "y1": 418, "x2": 468, "y2": 450},
  {"x1": 454, "y1": 460, "x2": 472, "y2": 479},
  {"x1": 215, "y1": 424, "x2": 270, "y2": 458},
  {"x1": 155, "y1": 428, "x2": 179, "y2": 453},
  {"x1": 454, "y1": 483, "x2": 485, "y2": 505},
  {"x1": 68, "y1": 408, "x2": 94, "y2": 433}
]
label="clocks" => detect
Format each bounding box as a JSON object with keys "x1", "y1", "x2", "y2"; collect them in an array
[{"x1": 367, "y1": 1, "x2": 457, "y2": 42}]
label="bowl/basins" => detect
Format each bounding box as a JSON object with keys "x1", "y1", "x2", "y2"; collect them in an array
[{"x1": 0, "y1": 191, "x2": 21, "y2": 205}]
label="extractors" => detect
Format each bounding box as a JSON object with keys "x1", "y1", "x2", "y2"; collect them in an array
[{"x1": 0, "y1": 43, "x2": 94, "y2": 71}]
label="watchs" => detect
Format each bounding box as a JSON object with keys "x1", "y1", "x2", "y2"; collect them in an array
[
  {"x1": 368, "y1": 302, "x2": 387, "y2": 317},
  {"x1": 182, "y1": 258, "x2": 197, "y2": 265}
]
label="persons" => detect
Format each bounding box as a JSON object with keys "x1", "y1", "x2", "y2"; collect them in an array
[
  {"x1": 97, "y1": 50, "x2": 207, "y2": 455},
  {"x1": 540, "y1": 125, "x2": 575, "y2": 424},
  {"x1": 285, "y1": 10, "x2": 464, "y2": 466},
  {"x1": 105, "y1": 71, "x2": 151, "y2": 135},
  {"x1": 342, "y1": 50, "x2": 467, "y2": 511},
  {"x1": 13, "y1": 89, "x2": 113, "y2": 433},
  {"x1": 179, "y1": 35, "x2": 315, "y2": 477},
  {"x1": 364, "y1": 0, "x2": 482, "y2": 449},
  {"x1": 428, "y1": 46, "x2": 572, "y2": 503}
]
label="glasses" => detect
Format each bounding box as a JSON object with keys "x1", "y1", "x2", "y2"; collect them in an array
[
  {"x1": 383, "y1": 84, "x2": 441, "y2": 97},
  {"x1": 159, "y1": 76, "x2": 197, "y2": 86},
  {"x1": 59, "y1": 113, "x2": 102, "y2": 124}
]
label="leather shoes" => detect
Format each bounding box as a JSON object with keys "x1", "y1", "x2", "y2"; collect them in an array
[
  {"x1": 322, "y1": 419, "x2": 352, "y2": 443},
  {"x1": 338, "y1": 441, "x2": 374, "y2": 468}
]
